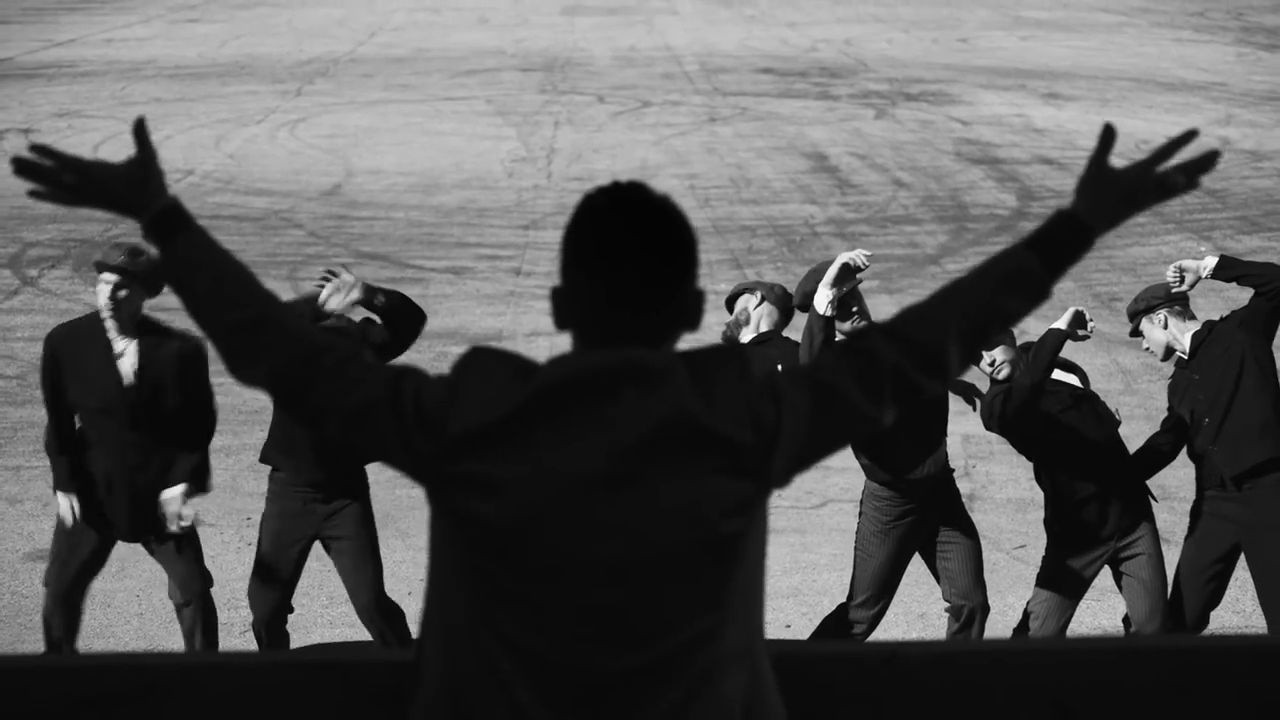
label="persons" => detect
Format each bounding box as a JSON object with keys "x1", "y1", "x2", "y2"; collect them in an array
[
  {"x1": 235, "y1": 262, "x2": 427, "y2": 649},
  {"x1": 725, "y1": 247, "x2": 990, "y2": 639},
  {"x1": 40, "y1": 244, "x2": 219, "y2": 653},
  {"x1": 9, "y1": 114, "x2": 1222, "y2": 720},
  {"x1": 970, "y1": 253, "x2": 1280, "y2": 639}
]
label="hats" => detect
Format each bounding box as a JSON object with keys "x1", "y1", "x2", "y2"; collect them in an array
[
  {"x1": 1125, "y1": 282, "x2": 1191, "y2": 339},
  {"x1": 794, "y1": 259, "x2": 863, "y2": 313},
  {"x1": 93, "y1": 240, "x2": 164, "y2": 299},
  {"x1": 723, "y1": 280, "x2": 796, "y2": 325}
]
